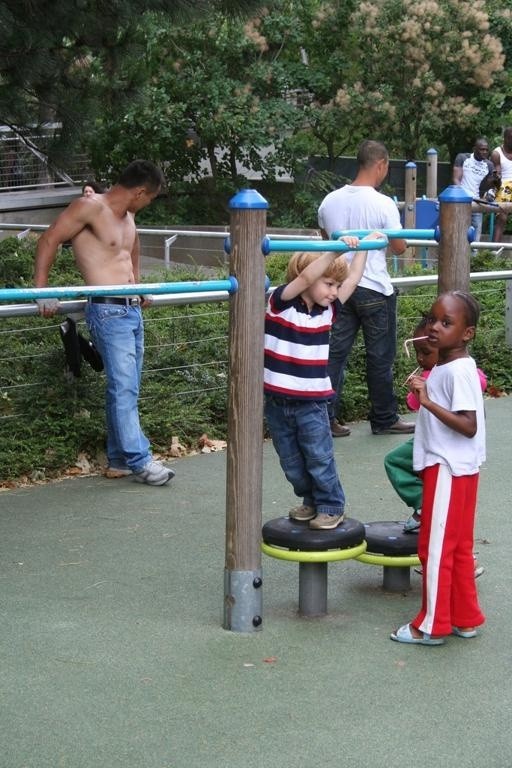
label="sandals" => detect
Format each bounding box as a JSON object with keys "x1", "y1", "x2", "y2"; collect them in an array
[
  {"x1": 402, "y1": 509, "x2": 422, "y2": 532},
  {"x1": 453, "y1": 624, "x2": 478, "y2": 638}
]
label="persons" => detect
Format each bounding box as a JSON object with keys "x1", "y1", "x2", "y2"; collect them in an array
[
  {"x1": 317, "y1": 139, "x2": 416, "y2": 438},
  {"x1": 260, "y1": 230, "x2": 390, "y2": 530},
  {"x1": 33, "y1": 157, "x2": 177, "y2": 488},
  {"x1": 487, "y1": 126, "x2": 511, "y2": 242},
  {"x1": 382, "y1": 311, "x2": 486, "y2": 579},
  {"x1": 390, "y1": 288, "x2": 485, "y2": 647},
  {"x1": 81, "y1": 181, "x2": 102, "y2": 198},
  {"x1": 451, "y1": 137, "x2": 501, "y2": 256}
]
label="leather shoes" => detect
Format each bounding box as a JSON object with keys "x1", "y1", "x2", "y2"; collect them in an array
[
  {"x1": 370, "y1": 419, "x2": 415, "y2": 434},
  {"x1": 330, "y1": 420, "x2": 351, "y2": 437}
]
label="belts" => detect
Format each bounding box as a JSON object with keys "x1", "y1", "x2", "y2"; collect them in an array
[{"x1": 87, "y1": 295, "x2": 142, "y2": 307}]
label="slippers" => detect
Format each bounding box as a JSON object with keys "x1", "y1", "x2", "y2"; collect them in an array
[{"x1": 390, "y1": 622, "x2": 445, "y2": 645}]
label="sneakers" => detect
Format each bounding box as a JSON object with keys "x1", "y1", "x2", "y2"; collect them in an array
[
  {"x1": 309, "y1": 513, "x2": 346, "y2": 529},
  {"x1": 104, "y1": 465, "x2": 133, "y2": 478},
  {"x1": 133, "y1": 462, "x2": 174, "y2": 486},
  {"x1": 289, "y1": 505, "x2": 314, "y2": 520}
]
